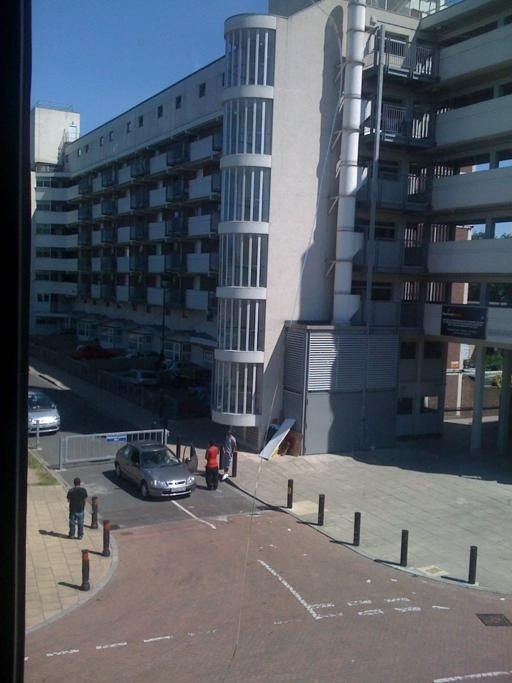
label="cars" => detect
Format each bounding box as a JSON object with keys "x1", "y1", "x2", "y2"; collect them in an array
[
  {"x1": 25, "y1": 386, "x2": 63, "y2": 438},
  {"x1": 110, "y1": 441, "x2": 200, "y2": 502},
  {"x1": 70, "y1": 341, "x2": 212, "y2": 392}
]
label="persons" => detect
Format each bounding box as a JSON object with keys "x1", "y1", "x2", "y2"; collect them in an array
[
  {"x1": 205, "y1": 439, "x2": 220, "y2": 490},
  {"x1": 65, "y1": 478, "x2": 88, "y2": 539},
  {"x1": 221, "y1": 431, "x2": 236, "y2": 481}
]
